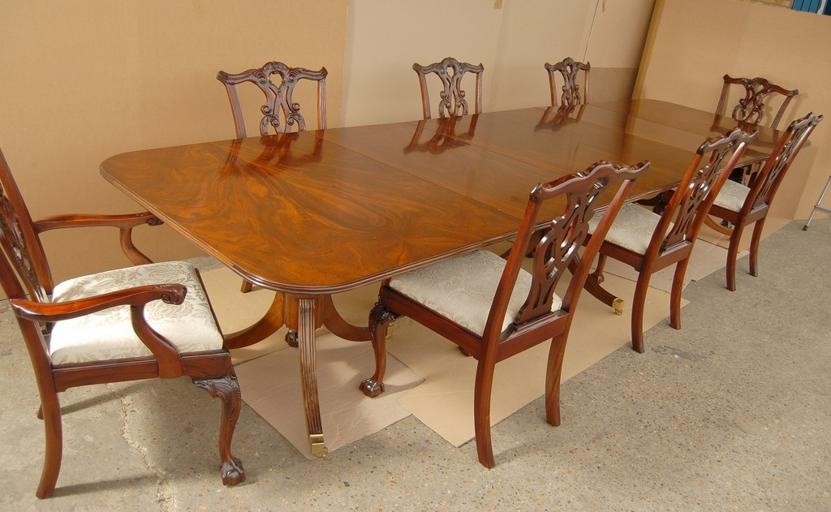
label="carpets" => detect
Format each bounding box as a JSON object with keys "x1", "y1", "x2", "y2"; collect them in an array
[{"x1": 200, "y1": 207, "x2": 794, "y2": 460}]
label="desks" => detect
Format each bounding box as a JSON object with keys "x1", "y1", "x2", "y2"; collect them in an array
[{"x1": 100, "y1": 99, "x2": 811, "y2": 458}]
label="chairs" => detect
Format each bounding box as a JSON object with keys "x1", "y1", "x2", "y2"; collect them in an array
[
  {"x1": 545, "y1": 58, "x2": 592, "y2": 105},
  {"x1": 660, "y1": 112, "x2": 824, "y2": 290},
  {"x1": 536, "y1": 104, "x2": 587, "y2": 129},
  {"x1": 405, "y1": 119, "x2": 479, "y2": 153},
  {"x1": 520, "y1": 128, "x2": 760, "y2": 352},
  {"x1": 712, "y1": 75, "x2": 800, "y2": 129},
  {"x1": 803, "y1": 174, "x2": 831, "y2": 231},
  {"x1": 413, "y1": 57, "x2": 485, "y2": 120},
  {"x1": 359, "y1": 158, "x2": 653, "y2": 469},
  {"x1": 218, "y1": 63, "x2": 328, "y2": 138},
  {"x1": 0, "y1": 150, "x2": 248, "y2": 498},
  {"x1": 224, "y1": 131, "x2": 325, "y2": 166}
]
